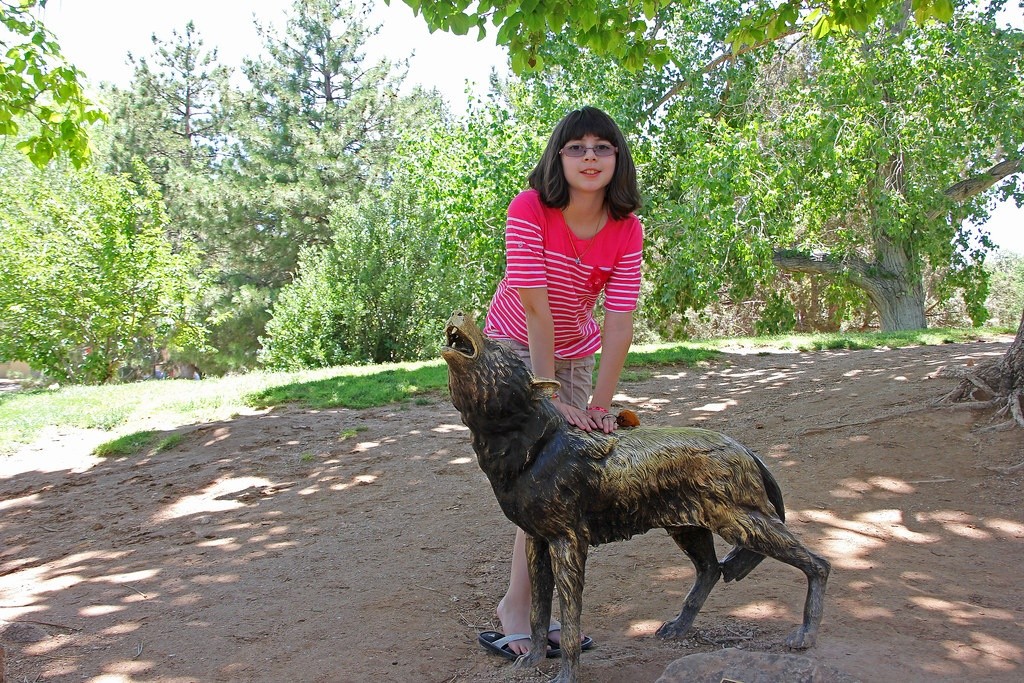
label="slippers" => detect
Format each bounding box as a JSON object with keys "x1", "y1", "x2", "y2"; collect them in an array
[
  {"x1": 478, "y1": 631, "x2": 560, "y2": 661},
  {"x1": 546, "y1": 624, "x2": 592, "y2": 651}
]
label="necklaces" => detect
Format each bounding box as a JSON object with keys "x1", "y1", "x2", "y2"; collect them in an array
[{"x1": 560, "y1": 207, "x2": 603, "y2": 264}]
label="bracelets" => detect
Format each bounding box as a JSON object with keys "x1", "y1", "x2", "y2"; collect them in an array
[
  {"x1": 589, "y1": 407, "x2": 610, "y2": 412},
  {"x1": 533, "y1": 378, "x2": 561, "y2": 398}
]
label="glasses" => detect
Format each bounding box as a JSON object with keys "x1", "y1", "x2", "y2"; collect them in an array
[{"x1": 559, "y1": 143, "x2": 619, "y2": 157}]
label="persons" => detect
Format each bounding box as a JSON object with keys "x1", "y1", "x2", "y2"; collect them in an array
[
  {"x1": 155, "y1": 363, "x2": 206, "y2": 381},
  {"x1": 479, "y1": 107, "x2": 643, "y2": 661}
]
